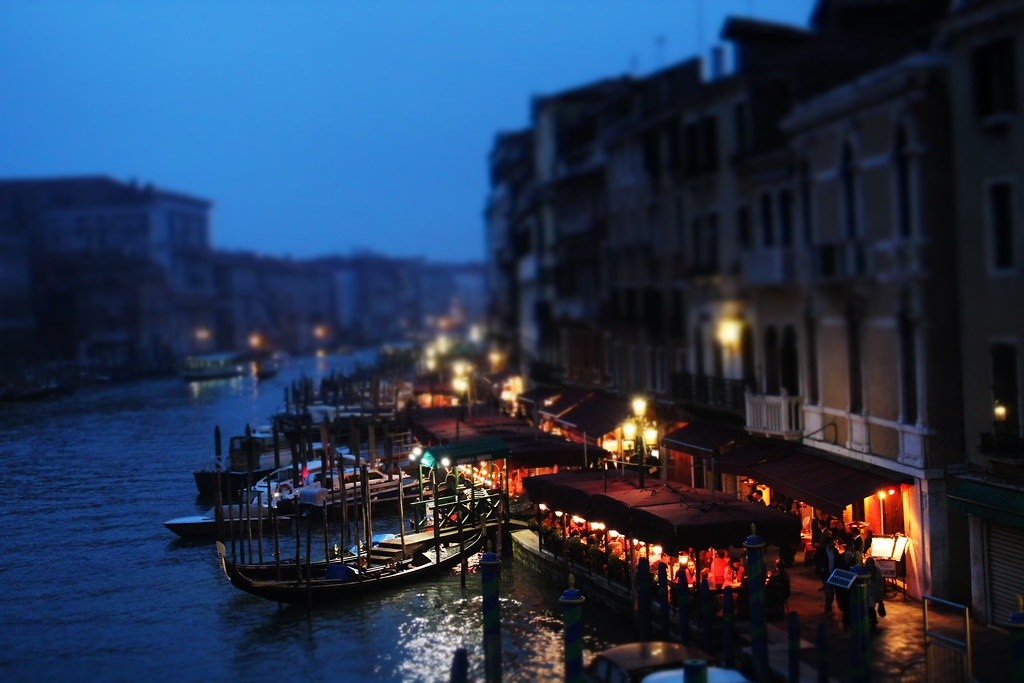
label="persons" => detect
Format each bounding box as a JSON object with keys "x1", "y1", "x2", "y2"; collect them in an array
[
  {"x1": 711, "y1": 550, "x2": 729, "y2": 578},
  {"x1": 812, "y1": 511, "x2": 885, "y2": 624},
  {"x1": 608, "y1": 537, "x2": 621, "y2": 553},
  {"x1": 725, "y1": 558, "x2": 744, "y2": 585},
  {"x1": 767, "y1": 497, "x2": 776, "y2": 510},
  {"x1": 685, "y1": 561, "x2": 696, "y2": 584},
  {"x1": 700, "y1": 551, "x2": 713, "y2": 572},
  {"x1": 544, "y1": 512, "x2": 553, "y2": 526},
  {"x1": 752, "y1": 490, "x2": 766, "y2": 507},
  {"x1": 774, "y1": 563, "x2": 791, "y2": 598},
  {"x1": 651, "y1": 553, "x2": 667, "y2": 571},
  {"x1": 570, "y1": 523, "x2": 584, "y2": 539}
]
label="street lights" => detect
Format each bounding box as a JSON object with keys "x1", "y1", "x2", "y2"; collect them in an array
[{"x1": 623, "y1": 390, "x2": 659, "y2": 489}]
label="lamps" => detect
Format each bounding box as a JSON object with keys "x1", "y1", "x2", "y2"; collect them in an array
[
  {"x1": 993, "y1": 398, "x2": 1008, "y2": 422},
  {"x1": 652, "y1": 544, "x2": 662, "y2": 555},
  {"x1": 678, "y1": 550, "x2": 690, "y2": 564}
]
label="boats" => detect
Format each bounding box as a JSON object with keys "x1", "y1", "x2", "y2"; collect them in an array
[
  {"x1": 216, "y1": 466, "x2": 491, "y2": 606},
  {"x1": 163, "y1": 345, "x2": 467, "y2": 541}
]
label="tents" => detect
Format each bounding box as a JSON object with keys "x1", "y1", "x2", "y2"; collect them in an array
[
  {"x1": 523, "y1": 467, "x2": 803, "y2": 618},
  {"x1": 415, "y1": 409, "x2": 608, "y2": 514}
]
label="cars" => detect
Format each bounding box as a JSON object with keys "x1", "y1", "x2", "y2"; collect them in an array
[{"x1": 592, "y1": 638, "x2": 748, "y2": 683}]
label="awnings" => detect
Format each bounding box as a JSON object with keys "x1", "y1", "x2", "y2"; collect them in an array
[
  {"x1": 717, "y1": 435, "x2": 913, "y2": 519},
  {"x1": 659, "y1": 424, "x2": 741, "y2": 488},
  {"x1": 517, "y1": 386, "x2": 566, "y2": 405},
  {"x1": 554, "y1": 391, "x2": 635, "y2": 467},
  {"x1": 538, "y1": 388, "x2": 595, "y2": 418}
]
